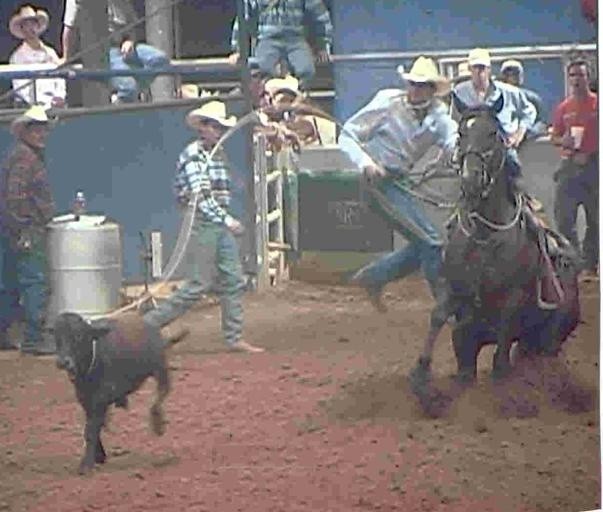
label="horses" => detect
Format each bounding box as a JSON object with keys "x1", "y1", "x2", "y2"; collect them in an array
[{"x1": 405, "y1": 90, "x2": 582, "y2": 418}]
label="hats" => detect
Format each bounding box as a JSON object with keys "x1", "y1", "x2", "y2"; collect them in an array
[
  {"x1": 394, "y1": 53, "x2": 453, "y2": 100},
  {"x1": 183, "y1": 99, "x2": 238, "y2": 131},
  {"x1": 11, "y1": 104, "x2": 60, "y2": 131},
  {"x1": 499, "y1": 58, "x2": 524, "y2": 86},
  {"x1": 6, "y1": 3, "x2": 51, "y2": 40},
  {"x1": 448, "y1": 61, "x2": 475, "y2": 84},
  {"x1": 262, "y1": 74, "x2": 302, "y2": 98},
  {"x1": 466, "y1": 46, "x2": 492, "y2": 70}
]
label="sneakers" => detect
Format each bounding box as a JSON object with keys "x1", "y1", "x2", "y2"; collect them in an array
[
  {"x1": 221, "y1": 342, "x2": 266, "y2": 355},
  {"x1": 0, "y1": 342, "x2": 56, "y2": 357},
  {"x1": 348, "y1": 268, "x2": 389, "y2": 315}
]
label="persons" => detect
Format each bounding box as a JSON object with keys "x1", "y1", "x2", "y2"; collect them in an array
[
  {"x1": 450, "y1": 46, "x2": 537, "y2": 148},
  {"x1": 336, "y1": 56, "x2": 459, "y2": 314},
  {"x1": 141, "y1": 100, "x2": 268, "y2": 356},
  {"x1": 262, "y1": 74, "x2": 306, "y2": 153},
  {"x1": 499, "y1": 59, "x2": 549, "y2": 140},
  {"x1": 8, "y1": 4, "x2": 68, "y2": 109},
  {"x1": 61, "y1": 0, "x2": 171, "y2": 104},
  {"x1": 1, "y1": 105, "x2": 58, "y2": 355},
  {"x1": 227, "y1": 0, "x2": 335, "y2": 79},
  {"x1": 550, "y1": 59, "x2": 599, "y2": 273}
]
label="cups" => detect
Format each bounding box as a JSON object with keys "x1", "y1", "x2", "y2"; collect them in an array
[{"x1": 569, "y1": 126, "x2": 585, "y2": 151}]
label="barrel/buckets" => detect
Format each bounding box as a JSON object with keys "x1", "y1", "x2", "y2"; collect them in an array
[{"x1": 43, "y1": 220, "x2": 125, "y2": 355}]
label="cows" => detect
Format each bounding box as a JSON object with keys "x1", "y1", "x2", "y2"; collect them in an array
[{"x1": 41, "y1": 311, "x2": 192, "y2": 476}]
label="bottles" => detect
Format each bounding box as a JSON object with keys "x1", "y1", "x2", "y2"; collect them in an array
[{"x1": 72, "y1": 192, "x2": 87, "y2": 221}]
